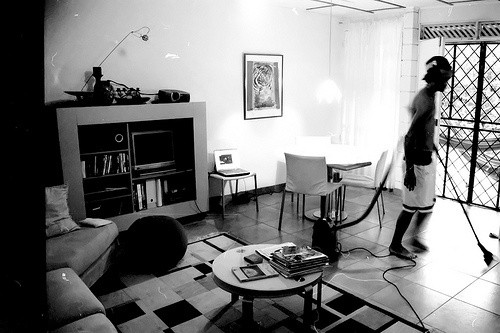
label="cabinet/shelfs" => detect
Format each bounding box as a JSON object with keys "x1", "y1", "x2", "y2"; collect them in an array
[{"x1": 56, "y1": 102, "x2": 209, "y2": 232}]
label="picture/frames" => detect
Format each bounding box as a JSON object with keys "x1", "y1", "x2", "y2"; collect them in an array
[{"x1": 244, "y1": 54, "x2": 283, "y2": 120}]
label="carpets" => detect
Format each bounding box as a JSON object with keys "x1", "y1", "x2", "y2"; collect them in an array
[{"x1": 96, "y1": 232, "x2": 432, "y2": 333}]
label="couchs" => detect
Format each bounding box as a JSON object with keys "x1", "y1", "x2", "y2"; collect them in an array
[{"x1": 44, "y1": 220, "x2": 119, "y2": 333}]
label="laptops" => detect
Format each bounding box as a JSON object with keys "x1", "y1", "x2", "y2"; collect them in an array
[{"x1": 214, "y1": 149, "x2": 250, "y2": 176}]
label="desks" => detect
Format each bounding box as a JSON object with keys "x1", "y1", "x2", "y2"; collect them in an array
[
  {"x1": 305, "y1": 162, "x2": 371, "y2": 223},
  {"x1": 211, "y1": 173, "x2": 259, "y2": 221}
]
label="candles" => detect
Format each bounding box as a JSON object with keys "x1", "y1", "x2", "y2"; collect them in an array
[{"x1": 94, "y1": 67, "x2": 101, "y2": 75}]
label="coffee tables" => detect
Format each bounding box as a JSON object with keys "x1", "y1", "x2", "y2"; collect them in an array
[{"x1": 213, "y1": 243, "x2": 323, "y2": 333}]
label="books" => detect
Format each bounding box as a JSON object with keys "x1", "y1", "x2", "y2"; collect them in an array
[
  {"x1": 271, "y1": 247, "x2": 328, "y2": 278},
  {"x1": 231, "y1": 262, "x2": 279, "y2": 282},
  {"x1": 81, "y1": 153, "x2": 129, "y2": 178}
]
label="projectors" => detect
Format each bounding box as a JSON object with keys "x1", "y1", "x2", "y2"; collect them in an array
[{"x1": 159, "y1": 89, "x2": 191, "y2": 103}]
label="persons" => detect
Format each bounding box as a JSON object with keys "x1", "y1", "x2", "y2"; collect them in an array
[{"x1": 389, "y1": 56, "x2": 452, "y2": 259}]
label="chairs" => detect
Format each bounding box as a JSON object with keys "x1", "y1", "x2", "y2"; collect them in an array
[{"x1": 278, "y1": 136, "x2": 393, "y2": 231}]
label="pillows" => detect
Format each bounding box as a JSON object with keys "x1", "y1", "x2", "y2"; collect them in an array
[{"x1": 45, "y1": 183, "x2": 81, "y2": 238}]
